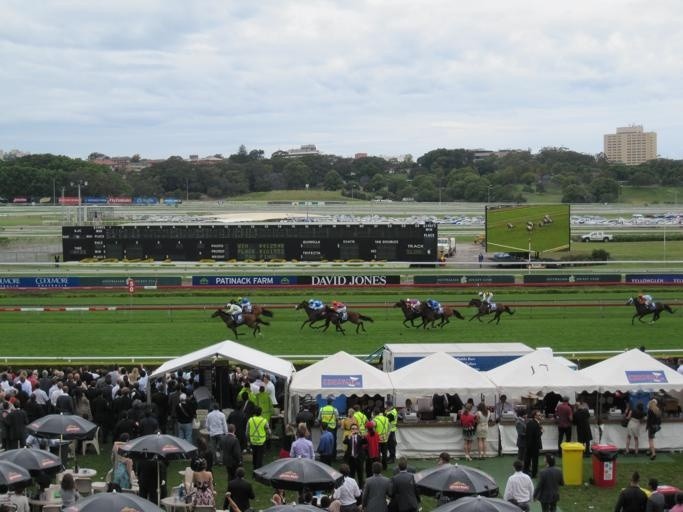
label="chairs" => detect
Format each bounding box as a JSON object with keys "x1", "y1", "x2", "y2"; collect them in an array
[{"x1": 0, "y1": 426, "x2": 216, "y2": 512}]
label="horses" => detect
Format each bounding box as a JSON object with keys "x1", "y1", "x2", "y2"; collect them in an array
[
  {"x1": 467, "y1": 298, "x2": 516, "y2": 325},
  {"x1": 416, "y1": 300, "x2": 465, "y2": 330},
  {"x1": 224, "y1": 299, "x2": 273, "y2": 318},
  {"x1": 626, "y1": 296, "x2": 673, "y2": 325},
  {"x1": 393, "y1": 299, "x2": 436, "y2": 329},
  {"x1": 212, "y1": 308, "x2": 270, "y2": 340},
  {"x1": 320, "y1": 304, "x2": 373, "y2": 336},
  {"x1": 296, "y1": 300, "x2": 334, "y2": 332}
]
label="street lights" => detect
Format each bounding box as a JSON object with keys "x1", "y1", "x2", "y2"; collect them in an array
[{"x1": 70, "y1": 180, "x2": 88, "y2": 226}]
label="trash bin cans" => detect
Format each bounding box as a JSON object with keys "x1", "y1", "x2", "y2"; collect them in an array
[
  {"x1": 560, "y1": 441, "x2": 586, "y2": 486},
  {"x1": 591, "y1": 443, "x2": 617, "y2": 488}
]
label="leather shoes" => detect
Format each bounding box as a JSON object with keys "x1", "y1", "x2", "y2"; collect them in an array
[{"x1": 386, "y1": 459, "x2": 394, "y2": 464}]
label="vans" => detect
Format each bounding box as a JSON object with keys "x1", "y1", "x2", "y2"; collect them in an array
[{"x1": 436, "y1": 236, "x2": 455, "y2": 257}]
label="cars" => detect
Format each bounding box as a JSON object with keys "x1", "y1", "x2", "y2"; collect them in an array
[
  {"x1": 122, "y1": 215, "x2": 218, "y2": 222},
  {"x1": 570, "y1": 215, "x2": 682, "y2": 228},
  {"x1": 310, "y1": 212, "x2": 486, "y2": 226}
]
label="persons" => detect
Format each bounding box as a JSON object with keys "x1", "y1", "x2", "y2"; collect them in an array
[
  {"x1": 638, "y1": 294, "x2": 655, "y2": 310},
  {"x1": 308, "y1": 299, "x2": 324, "y2": 312},
  {"x1": 479, "y1": 291, "x2": 494, "y2": 309},
  {"x1": 329, "y1": 300, "x2": 346, "y2": 318},
  {"x1": 405, "y1": 298, "x2": 421, "y2": 313},
  {"x1": 426, "y1": 298, "x2": 444, "y2": 315},
  {"x1": 478, "y1": 252, "x2": 483, "y2": 268},
  {"x1": 0, "y1": 363, "x2": 682, "y2": 512},
  {"x1": 222, "y1": 295, "x2": 253, "y2": 326}
]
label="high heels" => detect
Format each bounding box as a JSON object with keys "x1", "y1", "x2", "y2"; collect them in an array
[{"x1": 465, "y1": 454, "x2": 472, "y2": 461}]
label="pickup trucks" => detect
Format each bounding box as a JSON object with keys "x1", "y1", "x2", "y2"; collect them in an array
[{"x1": 580, "y1": 230, "x2": 614, "y2": 243}]
